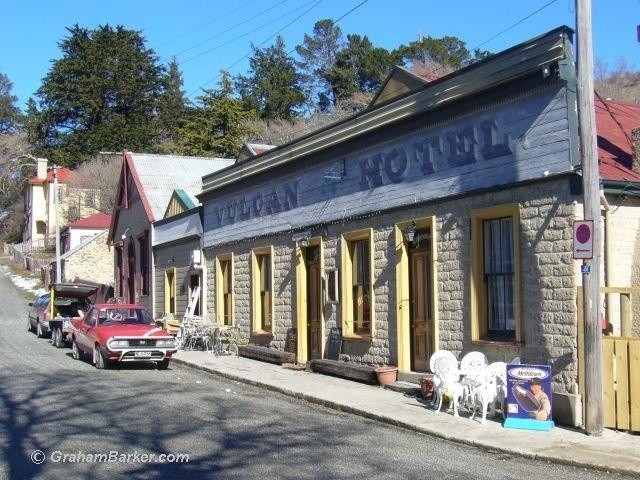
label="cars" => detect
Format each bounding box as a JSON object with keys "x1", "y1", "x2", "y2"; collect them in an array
[{"x1": 28, "y1": 283, "x2": 177, "y2": 370}]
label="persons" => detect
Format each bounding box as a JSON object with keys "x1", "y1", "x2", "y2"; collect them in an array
[
  {"x1": 108, "y1": 310, "x2": 122, "y2": 321},
  {"x1": 528, "y1": 377, "x2": 551, "y2": 420}
]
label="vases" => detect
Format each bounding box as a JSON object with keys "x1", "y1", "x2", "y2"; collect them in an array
[
  {"x1": 374, "y1": 366, "x2": 399, "y2": 388},
  {"x1": 418, "y1": 378, "x2": 434, "y2": 400}
]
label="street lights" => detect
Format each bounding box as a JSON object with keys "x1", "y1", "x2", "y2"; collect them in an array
[{"x1": 37, "y1": 158, "x2": 61, "y2": 283}]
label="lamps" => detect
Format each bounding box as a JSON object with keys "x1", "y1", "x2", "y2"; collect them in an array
[{"x1": 403, "y1": 223, "x2": 417, "y2": 242}]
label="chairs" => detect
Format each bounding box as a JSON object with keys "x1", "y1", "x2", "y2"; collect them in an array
[{"x1": 429, "y1": 351, "x2": 522, "y2": 423}]
